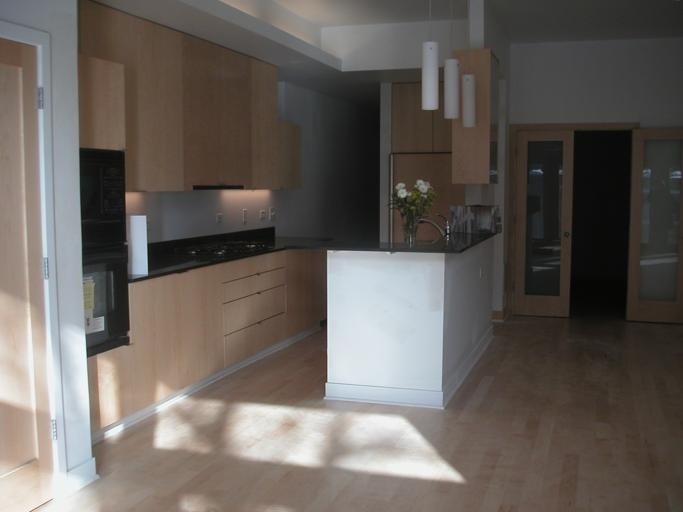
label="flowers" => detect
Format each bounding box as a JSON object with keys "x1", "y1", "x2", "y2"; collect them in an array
[{"x1": 387, "y1": 179, "x2": 440, "y2": 234}]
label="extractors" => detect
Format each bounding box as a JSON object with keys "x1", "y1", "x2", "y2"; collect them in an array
[{"x1": 193, "y1": 185, "x2": 244, "y2": 190}]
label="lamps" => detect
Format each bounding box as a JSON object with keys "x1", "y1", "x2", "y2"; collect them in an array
[{"x1": 419, "y1": 0, "x2": 478, "y2": 130}]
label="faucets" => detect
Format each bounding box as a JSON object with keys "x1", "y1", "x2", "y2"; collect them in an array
[{"x1": 416, "y1": 213, "x2": 451, "y2": 239}]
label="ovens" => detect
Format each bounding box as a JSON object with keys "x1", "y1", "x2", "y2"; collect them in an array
[{"x1": 76, "y1": 146, "x2": 130, "y2": 361}]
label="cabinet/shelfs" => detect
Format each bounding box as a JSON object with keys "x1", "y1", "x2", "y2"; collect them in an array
[
  {"x1": 396, "y1": 151, "x2": 463, "y2": 240},
  {"x1": 276, "y1": 121, "x2": 302, "y2": 193},
  {"x1": 87, "y1": 277, "x2": 155, "y2": 440},
  {"x1": 76, "y1": 2, "x2": 184, "y2": 195},
  {"x1": 393, "y1": 85, "x2": 458, "y2": 154},
  {"x1": 285, "y1": 241, "x2": 332, "y2": 340},
  {"x1": 184, "y1": 33, "x2": 256, "y2": 189},
  {"x1": 253, "y1": 58, "x2": 279, "y2": 190},
  {"x1": 452, "y1": 51, "x2": 501, "y2": 186},
  {"x1": 77, "y1": 53, "x2": 126, "y2": 153},
  {"x1": 154, "y1": 262, "x2": 224, "y2": 410},
  {"x1": 224, "y1": 247, "x2": 286, "y2": 374}
]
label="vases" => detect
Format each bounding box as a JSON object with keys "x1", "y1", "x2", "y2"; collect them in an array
[{"x1": 405, "y1": 226, "x2": 416, "y2": 242}]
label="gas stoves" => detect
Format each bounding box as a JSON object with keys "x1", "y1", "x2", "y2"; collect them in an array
[{"x1": 182, "y1": 240, "x2": 278, "y2": 262}]
